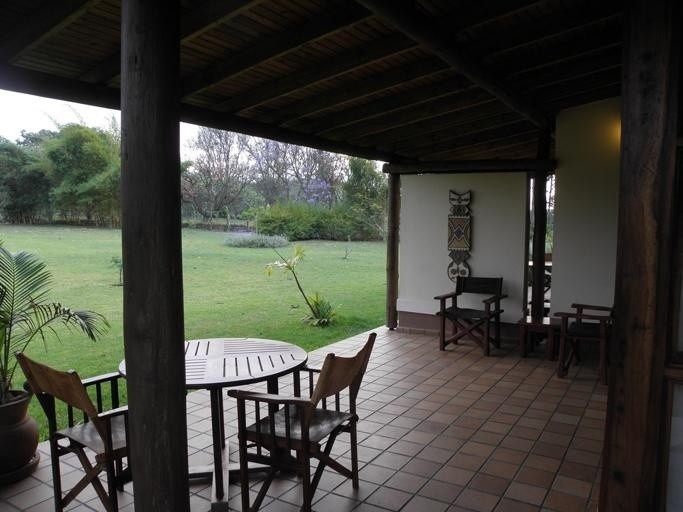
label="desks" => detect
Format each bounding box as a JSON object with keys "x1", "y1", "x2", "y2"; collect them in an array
[{"x1": 115, "y1": 336, "x2": 309, "y2": 511}]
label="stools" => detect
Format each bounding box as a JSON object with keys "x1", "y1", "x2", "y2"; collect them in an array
[{"x1": 519, "y1": 315, "x2": 565, "y2": 360}]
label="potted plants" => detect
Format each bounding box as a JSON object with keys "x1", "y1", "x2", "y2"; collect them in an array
[{"x1": 0, "y1": 242, "x2": 112, "y2": 488}]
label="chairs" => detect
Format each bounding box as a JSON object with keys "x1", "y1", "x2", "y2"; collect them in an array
[
  {"x1": 551, "y1": 303, "x2": 613, "y2": 387},
  {"x1": 227, "y1": 330, "x2": 379, "y2": 512},
  {"x1": 434, "y1": 274, "x2": 508, "y2": 359},
  {"x1": 12, "y1": 352, "x2": 135, "y2": 512}
]
